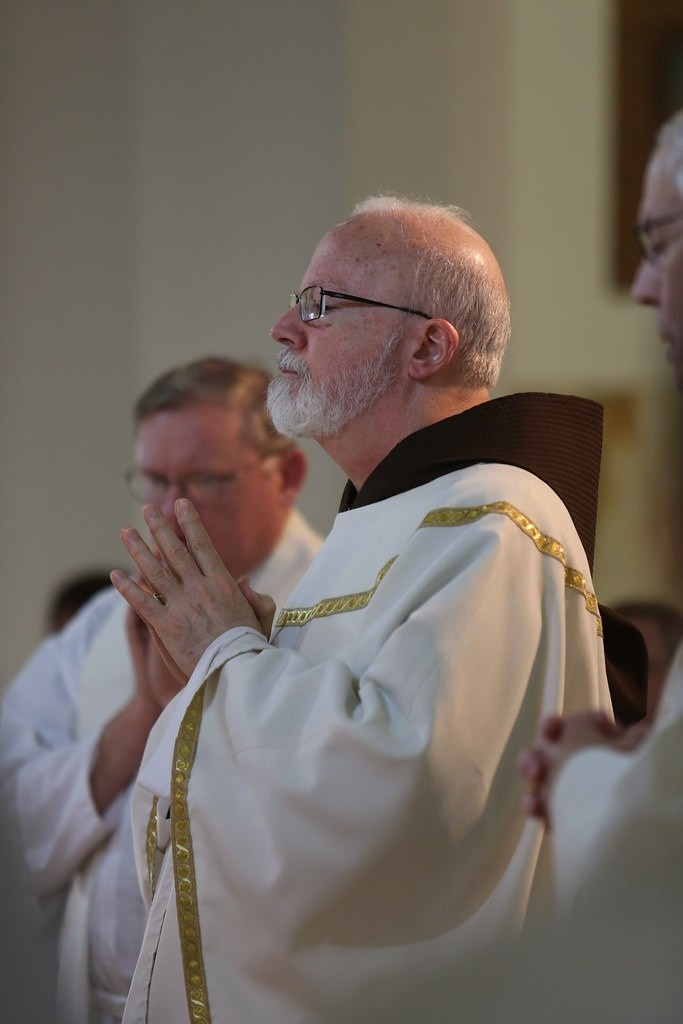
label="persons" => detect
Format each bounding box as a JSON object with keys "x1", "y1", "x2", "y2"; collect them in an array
[
  {"x1": 518, "y1": 108, "x2": 683, "y2": 1021},
  {"x1": 0, "y1": 354, "x2": 387, "y2": 1024},
  {"x1": 44, "y1": 566, "x2": 130, "y2": 643},
  {"x1": 109, "y1": 196, "x2": 617, "y2": 1021}
]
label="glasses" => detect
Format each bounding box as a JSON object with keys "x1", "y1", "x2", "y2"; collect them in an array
[
  {"x1": 287, "y1": 284, "x2": 431, "y2": 323},
  {"x1": 636, "y1": 214, "x2": 683, "y2": 265},
  {"x1": 125, "y1": 455, "x2": 269, "y2": 511}
]
